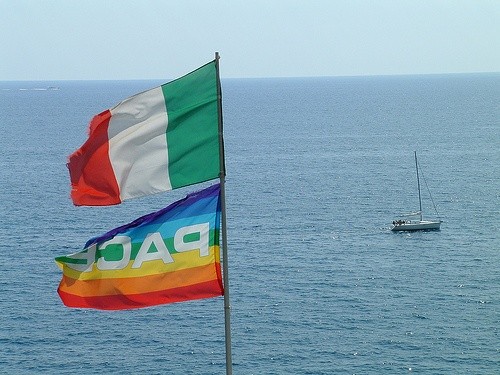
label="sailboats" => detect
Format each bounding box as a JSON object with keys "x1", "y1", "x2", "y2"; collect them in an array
[{"x1": 391, "y1": 151, "x2": 443, "y2": 232}]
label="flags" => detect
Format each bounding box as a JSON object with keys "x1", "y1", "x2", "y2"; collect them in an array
[
  {"x1": 53, "y1": 183, "x2": 225, "y2": 312},
  {"x1": 67, "y1": 57, "x2": 223, "y2": 207}
]
